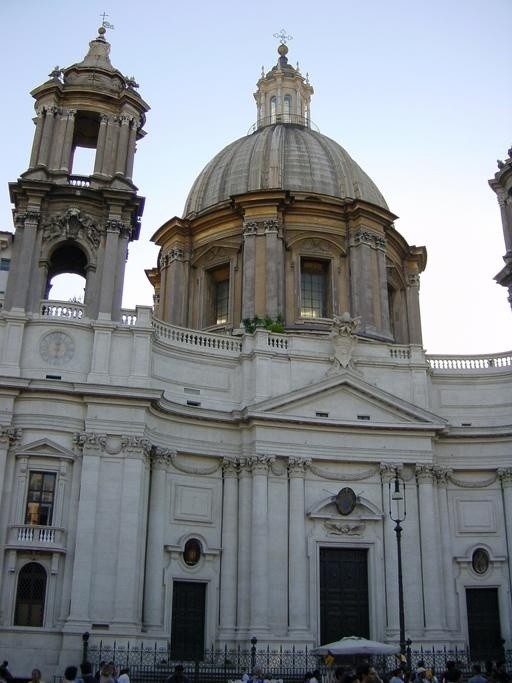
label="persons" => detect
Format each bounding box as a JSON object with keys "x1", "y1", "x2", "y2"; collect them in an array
[{"x1": 0, "y1": 657, "x2": 512, "y2": 683}]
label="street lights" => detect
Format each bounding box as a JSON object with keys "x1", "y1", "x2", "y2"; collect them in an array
[{"x1": 388, "y1": 468, "x2": 412, "y2": 652}]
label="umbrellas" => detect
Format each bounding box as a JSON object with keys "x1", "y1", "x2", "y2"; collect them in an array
[{"x1": 307, "y1": 634, "x2": 403, "y2": 656}]
